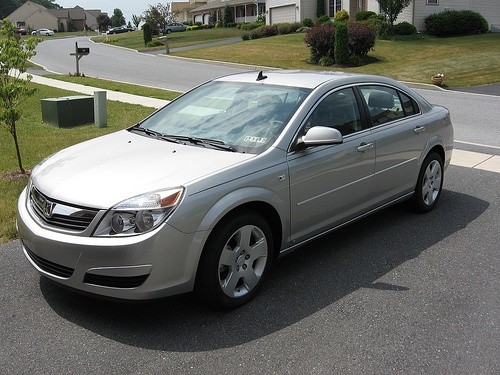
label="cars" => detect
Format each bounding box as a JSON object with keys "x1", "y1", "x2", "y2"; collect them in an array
[
  {"x1": 95, "y1": 24, "x2": 136, "y2": 35},
  {"x1": 31, "y1": 28, "x2": 54, "y2": 37},
  {"x1": 158, "y1": 22, "x2": 186, "y2": 33},
  {"x1": 15, "y1": 70, "x2": 455, "y2": 315},
  {"x1": 15, "y1": 26, "x2": 28, "y2": 35}
]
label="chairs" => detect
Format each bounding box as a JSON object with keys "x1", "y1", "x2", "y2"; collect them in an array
[{"x1": 368, "y1": 92, "x2": 396, "y2": 126}]
